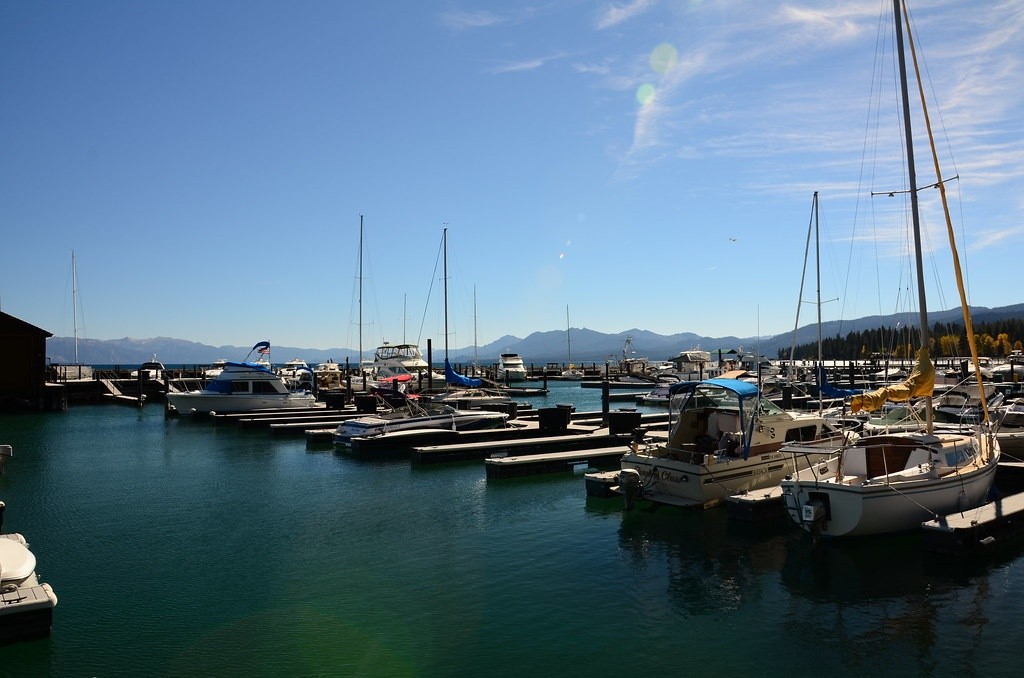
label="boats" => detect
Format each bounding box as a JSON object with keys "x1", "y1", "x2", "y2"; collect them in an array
[
  {"x1": 129, "y1": 355, "x2": 166, "y2": 379},
  {"x1": 609, "y1": 355, "x2": 784, "y2": 382},
  {"x1": 716, "y1": 370, "x2": 775, "y2": 395},
  {"x1": 610, "y1": 377, "x2": 861, "y2": 514},
  {"x1": 318, "y1": 344, "x2": 447, "y2": 388},
  {"x1": 168, "y1": 361, "x2": 318, "y2": 420},
  {"x1": 333, "y1": 394, "x2": 510, "y2": 456},
  {"x1": 498, "y1": 353, "x2": 528, "y2": 381},
  {"x1": 871, "y1": 351, "x2": 1024, "y2": 381},
  {"x1": 561, "y1": 368, "x2": 584, "y2": 378},
  {"x1": 201, "y1": 359, "x2": 227, "y2": 377},
  {"x1": 793, "y1": 383, "x2": 1024, "y2": 428},
  {"x1": 778, "y1": 428, "x2": 1001, "y2": 550}
]
